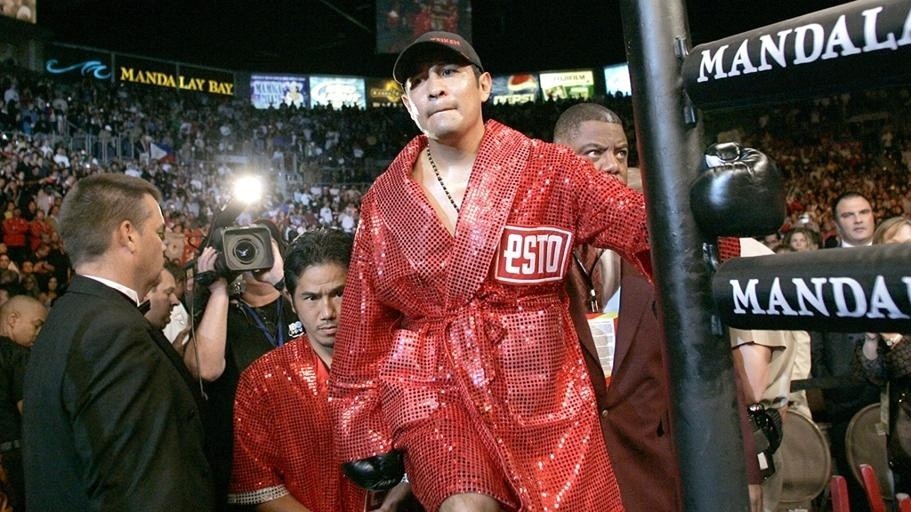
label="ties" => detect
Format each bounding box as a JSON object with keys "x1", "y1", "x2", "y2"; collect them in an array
[{"x1": 570, "y1": 241, "x2": 603, "y2": 312}]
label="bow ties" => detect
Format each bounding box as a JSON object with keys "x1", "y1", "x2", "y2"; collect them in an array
[{"x1": 137, "y1": 299, "x2": 151, "y2": 316}]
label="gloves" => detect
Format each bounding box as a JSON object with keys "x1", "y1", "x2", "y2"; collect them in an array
[{"x1": 690, "y1": 140, "x2": 787, "y2": 237}]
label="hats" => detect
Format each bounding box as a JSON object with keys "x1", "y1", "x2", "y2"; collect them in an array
[{"x1": 392, "y1": 30, "x2": 484, "y2": 94}]
